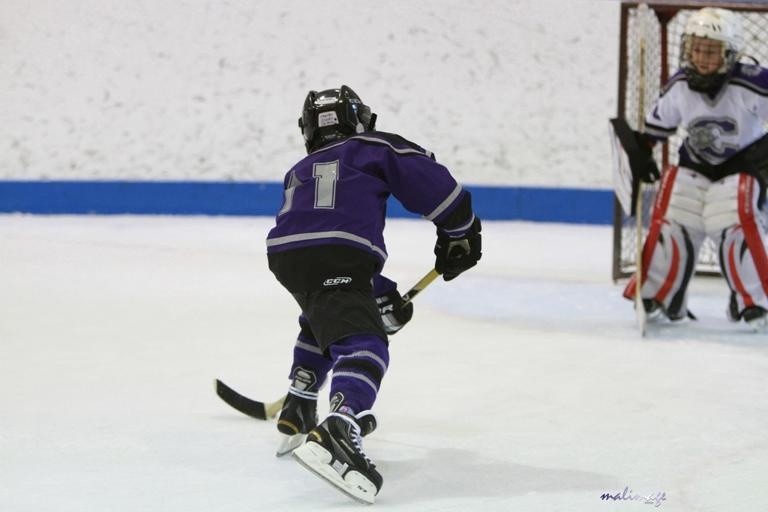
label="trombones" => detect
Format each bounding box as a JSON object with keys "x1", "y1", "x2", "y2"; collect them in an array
[{"x1": 217, "y1": 246, "x2": 466, "y2": 420}]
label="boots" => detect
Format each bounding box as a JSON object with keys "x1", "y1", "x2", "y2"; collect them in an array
[
  {"x1": 277, "y1": 367, "x2": 319, "y2": 434},
  {"x1": 307, "y1": 391, "x2": 383, "y2": 496}
]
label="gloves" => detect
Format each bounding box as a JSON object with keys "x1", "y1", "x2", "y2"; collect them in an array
[
  {"x1": 433, "y1": 217, "x2": 482, "y2": 281},
  {"x1": 373, "y1": 287, "x2": 413, "y2": 334}
]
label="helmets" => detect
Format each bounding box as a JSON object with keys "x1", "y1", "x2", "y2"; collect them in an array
[
  {"x1": 678, "y1": 7, "x2": 745, "y2": 93},
  {"x1": 300, "y1": 85, "x2": 377, "y2": 155}
]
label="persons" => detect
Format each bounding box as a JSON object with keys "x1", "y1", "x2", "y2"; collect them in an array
[
  {"x1": 266, "y1": 86, "x2": 481, "y2": 497},
  {"x1": 609, "y1": 5, "x2": 768, "y2": 328}
]
